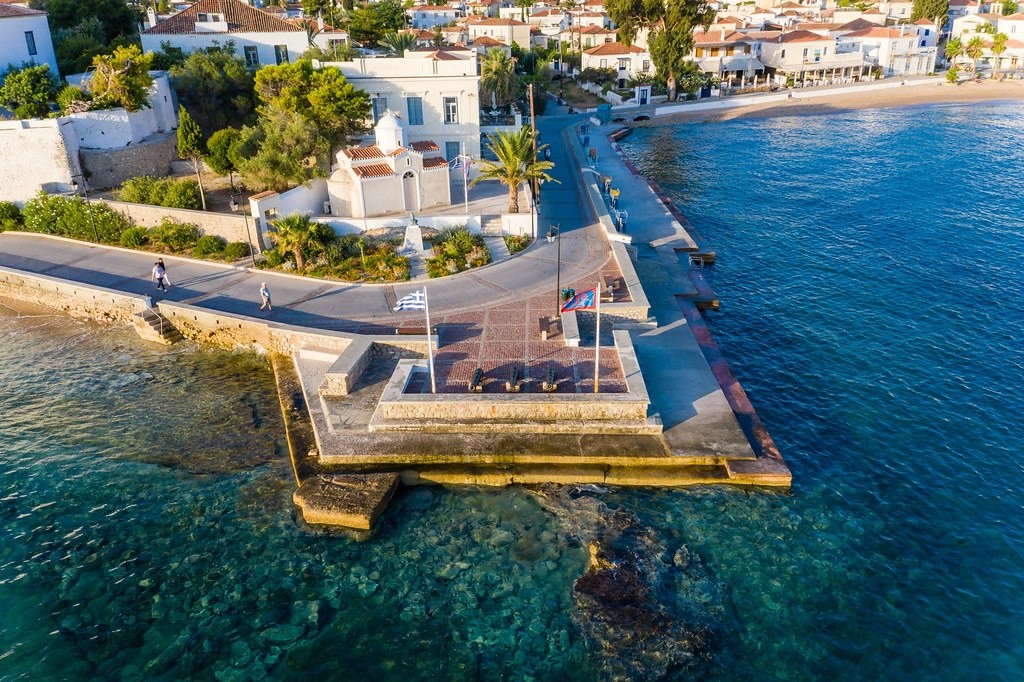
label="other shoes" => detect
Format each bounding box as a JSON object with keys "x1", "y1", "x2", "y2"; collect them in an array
[
  {"x1": 164, "y1": 289, "x2": 168, "y2": 293},
  {"x1": 157, "y1": 287, "x2": 162, "y2": 290},
  {"x1": 260, "y1": 307, "x2": 264, "y2": 311},
  {"x1": 169, "y1": 284, "x2": 174, "y2": 286}
]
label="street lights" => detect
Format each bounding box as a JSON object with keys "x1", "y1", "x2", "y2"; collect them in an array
[
  {"x1": 228, "y1": 187, "x2": 256, "y2": 267},
  {"x1": 544, "y1": 221, "x2": 561, "y2": 317},
  {"x1": 69, "y1": 173, "x2": 101, "y2": 242}
]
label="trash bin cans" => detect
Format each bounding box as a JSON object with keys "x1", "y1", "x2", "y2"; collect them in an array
[
  {"x1": 589, "y1": 148, "x2": 596, "y2": 163},
  {"x1": 561, "y1": 289, "x2": 568, "y2": 300},
  {"x1": 583, "y1": 135, "x2": 590, "y2": 147},
  {"x1": 569, "y1": 288, "x2": 575, "y2": 297},
  {"x1": 581, "y1": 125, "x2": 586, "y2": 133}
]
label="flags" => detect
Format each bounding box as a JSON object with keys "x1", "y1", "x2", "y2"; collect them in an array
[
  {"x1": 462, "y1": 161, "x2": 470, "y2": 177},
  {"x1": 392, "y1": 289, "x2": 426, "y2": 311},
  {"x1": 560, "y1": 287, "x2": 596, "y2": 313}
]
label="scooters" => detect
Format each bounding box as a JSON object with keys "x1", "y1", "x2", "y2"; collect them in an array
[{"x1": 544, "y1": 148, "x2": 553, "y2": 161}]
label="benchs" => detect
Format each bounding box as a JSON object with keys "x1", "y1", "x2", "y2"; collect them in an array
[
  {"x1": 396, "y1": 327, "x2": 437, "y2": 335},
  {"x1": 539, "y1": 316, "x2": 550, "y2": 342},
  {"x1": 600, "y1": 292, "x2": 614, "y2": 302},
  {"x1": 604, "y1": 275, "x2": 615, "y2": 293}
]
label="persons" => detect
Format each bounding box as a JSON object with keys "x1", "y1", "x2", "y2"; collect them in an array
[
  {"x1": 152, "y1": 262, "x2": 168, "y2": 292},
  {"x1": 259, "y1": 283, "x2": 272, "y2": 312},
  {"x1": 158, "y1": 258, "x2": 174, "y2": 287}
]
